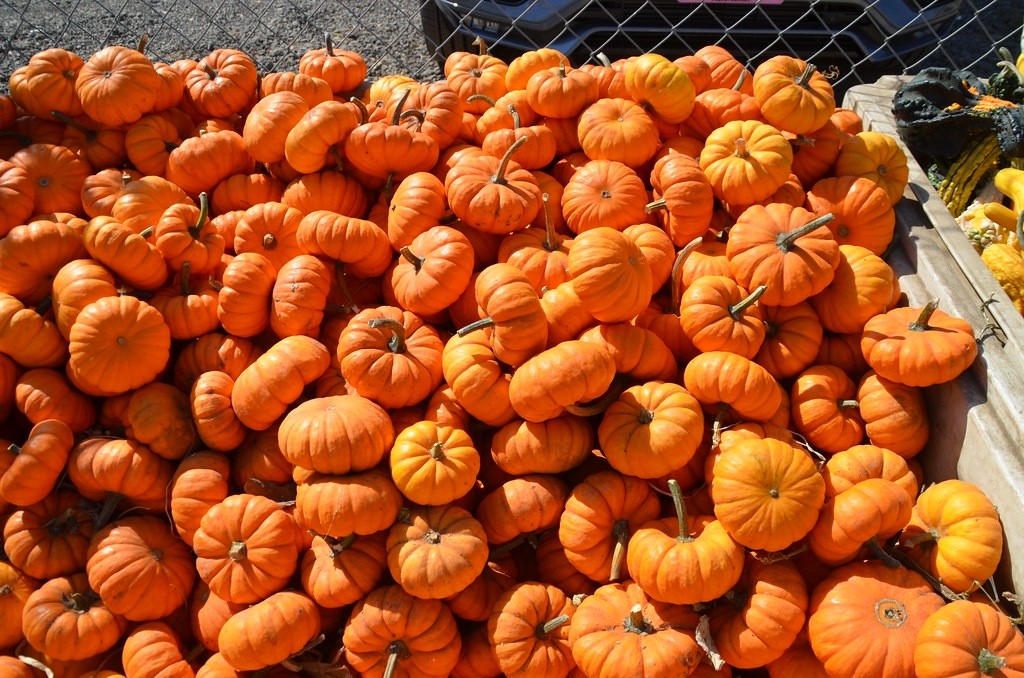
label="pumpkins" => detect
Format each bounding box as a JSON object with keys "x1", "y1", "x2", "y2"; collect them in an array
[{"x1": 0, "y1": 30, "x2": 1024, "y2": 678}]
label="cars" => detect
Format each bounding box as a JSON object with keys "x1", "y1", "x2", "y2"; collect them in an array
[{"x1": 419, "y1": 0, "x2": 1024, "y2": 106}]
